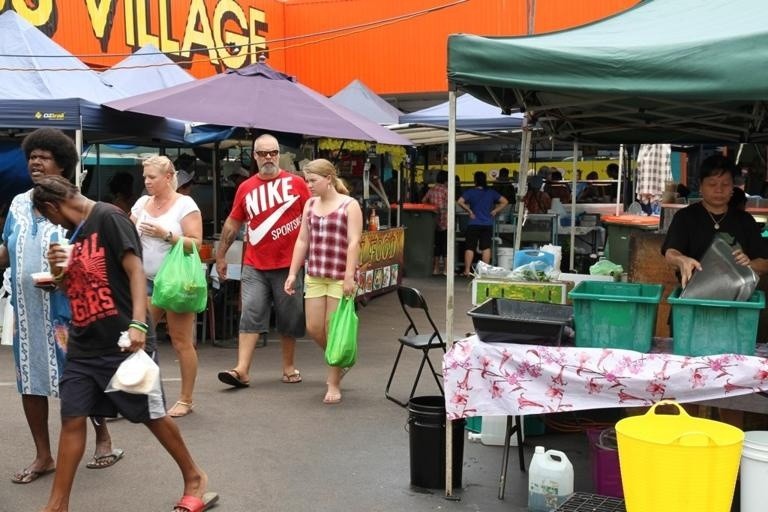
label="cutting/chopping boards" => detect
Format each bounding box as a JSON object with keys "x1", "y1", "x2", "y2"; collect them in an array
[{"x1": 512, "y1": 248, "x2": 555, "y2": 275}]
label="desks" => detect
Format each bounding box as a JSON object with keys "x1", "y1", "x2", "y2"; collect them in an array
[
  {"x1": 199, "y1": 255, "x2": 270, "y2": 345},
  {"x1": 441, "y1": 334, "x2": 768, "y2": 500},
  {"x1": 355, "y1": 227, "x2": 408, "y2": 302},
  {"x1": 363, "y1": 180, "x2": 633, "y2": 273}
]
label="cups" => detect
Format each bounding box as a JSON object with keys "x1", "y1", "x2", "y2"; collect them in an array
[
  {"x1": 55, "y1": 244, "x2": 73, "y2": 267},
  {"x1": 199, "y1": 245, "x2": 212, "y2": 259},
  {"x1": 367, "y1": 224, "x2": 376, "y2": 231}
]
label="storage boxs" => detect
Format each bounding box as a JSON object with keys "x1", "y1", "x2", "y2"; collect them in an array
[
  {"x1": 666, "y1": 284, "x2": 766, "y2": 356},
  {"x1": 679, "y1": 237, "x2": 760, "y2": 302},
  {"x1": 471, "y1": 278, "x2": 575, "y2": 306},
  {"x1": 567, "y1": 280, "x2": 665, "y2": 353}
]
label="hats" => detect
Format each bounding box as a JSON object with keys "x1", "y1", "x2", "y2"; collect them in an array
[{"x1": 176, "y1": 169, "x2": 195, "y2": 191}]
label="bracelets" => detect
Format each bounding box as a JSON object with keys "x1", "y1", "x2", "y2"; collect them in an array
[{"x1": 164, "y1": 232, "x2": 172, "y2": 242}]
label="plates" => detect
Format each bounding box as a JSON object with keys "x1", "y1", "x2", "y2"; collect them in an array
[{"x1": 30, "y1": 272, "x2": 52, "y2": 282}]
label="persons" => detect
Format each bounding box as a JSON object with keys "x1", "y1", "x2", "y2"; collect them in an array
[
  {"x1": 0, "y1": 127, "x2": 125, "y2": 484},
  {"x1": 284, "y1": 158, "x2": 363, "y2": 405},
  {"x1": 174, "y1": 169, "x2": 229, "y2": 223},
  {"x1": 129, "y1": 156, "x2": 203, "y2": 416},
  {"x1": 421, "y1": 163, "x2": 623, "y2": 279},
  {"x1": 216, "y1": 134, "x2": 312, "y2": 387},
  {"x1": 110, "y1": 172, "x2": 135, "y2": 212},
  {"x1": 661, "y1": 155, "x2": 768, "y2": 344},
  {"x1": 32, "y1": 175, "x2": 219, "y2": 512}
]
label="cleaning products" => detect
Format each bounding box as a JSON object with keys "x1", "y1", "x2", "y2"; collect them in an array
[{"x1": 369, "y1": 209, "x2": 376, "y2": 224}]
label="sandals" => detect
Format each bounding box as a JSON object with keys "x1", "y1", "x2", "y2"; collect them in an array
[{"x1": 167, "y1": 399, "x2": 194, "y2": 418}]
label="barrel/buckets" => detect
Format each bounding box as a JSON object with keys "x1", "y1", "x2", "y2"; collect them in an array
[
  {"x1": 528, "y1": 445, "x2": 574, "y2": 512},
  {"x1": 496, "y1": 247, "x2": 514, "y2": 270},
  {"x1": 740, "y1": 430, "x2": 768, "y2": 512},
  {"x1": 404, "y1": 396, "x2": 467, "y2": 489},
  {"x1": 480, "y1": 416, "x2": 523, "y2": 445}
]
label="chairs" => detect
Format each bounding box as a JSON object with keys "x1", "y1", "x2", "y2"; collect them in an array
[
  {"x1": 164, "y1": 291, "x2": 217, "y2": 345},
  {"x1": 385, "y1": 286, "x2": 465, "y2": 407}
]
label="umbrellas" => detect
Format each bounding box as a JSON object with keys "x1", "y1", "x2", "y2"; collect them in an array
[{"x1": 101, "y1": 52, "x2": 415, "y2": 234}]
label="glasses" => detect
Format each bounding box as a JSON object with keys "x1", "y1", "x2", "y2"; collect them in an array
[{"x1": 256, "y1": 149, "x2": 280, "y2": 157}]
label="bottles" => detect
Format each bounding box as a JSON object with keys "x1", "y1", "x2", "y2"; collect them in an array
[
  {"x1": 588, "y1": 245, "x2": 606, "y2": 273},
  {"x1": 370, "y1": 208, "x2": 380, "y2": 231}
]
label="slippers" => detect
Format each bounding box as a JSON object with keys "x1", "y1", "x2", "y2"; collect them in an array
[
  {"x1": 324, "y1": 392, "x2": 341, "y2": 404},
  {"x1": 86, "y1": 448, "x2": 124, "y2": 469},
  {"x1": 12, "y1": 462, "x2": 56, "y2": 483},
  {"x1": 218, "y1": 369, "x2": 250, "y2": 388},
  {"x1": 174, "y1": 492, "x2": 221, "y2": 511},
  {"x1": 283, "y1": 369, "x2": 302, "y2": 384}
]
